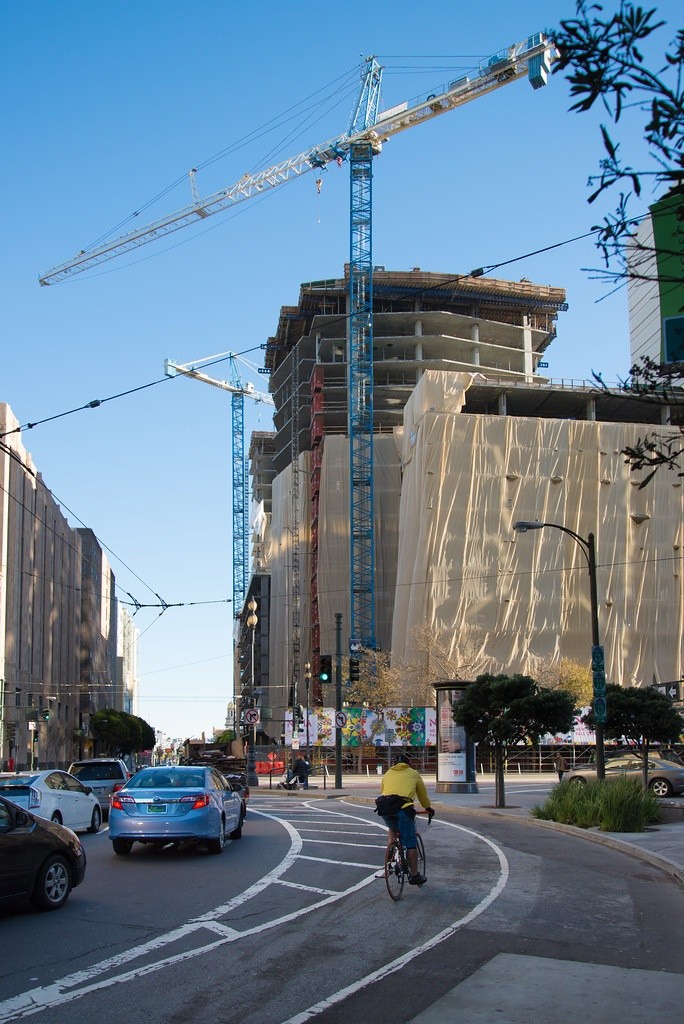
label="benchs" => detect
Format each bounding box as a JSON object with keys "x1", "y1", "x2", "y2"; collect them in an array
[{"x1": 140, "y1": 780, "x2": 202, "y2": 787}]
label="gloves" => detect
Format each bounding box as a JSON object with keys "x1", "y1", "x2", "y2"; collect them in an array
[{"x1": 425, "y1": 807, "x2": 435, "y2": 816}]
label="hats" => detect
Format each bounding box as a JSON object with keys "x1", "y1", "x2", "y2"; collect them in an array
[{"x1": 394, "y1": 754, "x2": 411, "y2": 767}]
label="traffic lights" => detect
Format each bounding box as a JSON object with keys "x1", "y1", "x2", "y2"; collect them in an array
[
  {"x1": 43, "y1": 709, "x2": 49, "y2": 720},
  {"x1": 298, "y1": 720, "x2": 304, "y2": 732},
  {"x1": 318, "y1": 654, "x2": 332, "y2": 683},
  {"x1": 295, "y1": 705, "x2": 301, "y2": 718},
  {"x1": 348, "y1": 657, "x2": 360, "y2": 683},
  {"x1": 33, "y1": 730, "x2": 39, "y2": 742}
]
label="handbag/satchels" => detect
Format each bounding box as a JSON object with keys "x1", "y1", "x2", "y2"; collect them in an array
[{"x1": 375, "y1": 795, "x2": 409, "y2": 816}]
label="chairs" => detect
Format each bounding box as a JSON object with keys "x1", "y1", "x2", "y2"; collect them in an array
[{"x1": 152, "y1": 776, "x2": 171, "y2": 784}]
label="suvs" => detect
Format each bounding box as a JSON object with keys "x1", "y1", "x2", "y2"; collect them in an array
[{"x1": 67, "y1": 756, "x2": 132, "y2": 809}]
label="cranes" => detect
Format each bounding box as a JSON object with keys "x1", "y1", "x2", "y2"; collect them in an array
[
  {"x1": 164, "y1": 351, "x2": 276, "y2": 618},
  {"x1": 38, "y1": 29, "x2": 561, "y2": 672}
]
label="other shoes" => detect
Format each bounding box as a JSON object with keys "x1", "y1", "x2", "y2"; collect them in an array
[
  {"x1": 388, "y1": 848, "x2": 395, "y2": 861},
  {"x1": 408, "y1": 872, "x2": 427, "y2": 885}
]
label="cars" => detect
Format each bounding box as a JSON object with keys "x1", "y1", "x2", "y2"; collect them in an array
[
  {"x1": 0, "y1": 769, "x2": 103, "y2": 833},
  {"x1": 0, "y1": 796, "x2": 87, "y2": 916},
  {"x1": 108, "y1": 765, "x2": 247, "y2": 854},
  {"x1": 559, "y1": 757, "x2": 684, "y2": 798}
]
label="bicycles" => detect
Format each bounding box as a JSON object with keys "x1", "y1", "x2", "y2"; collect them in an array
[{"x1": 374, "y1": 807, "x2": 431, "y2": 901}]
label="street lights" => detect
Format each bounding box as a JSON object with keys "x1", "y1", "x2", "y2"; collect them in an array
[
  {"x1": 247, "y1": 594, "x2": 259, "y2": 787},
  {"x1": 304, "y1": 660, "x2": 312, "y2": 761},
  {"x1": 88, "y1": 720, "x2": 108, "y2": 728},
  {"x1": 512, "y1": 519, "x2": 606, "y2": 780}
]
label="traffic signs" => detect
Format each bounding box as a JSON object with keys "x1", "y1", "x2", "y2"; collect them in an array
[{"x1": 665, "y1": 684, "x2": 679, "y2": 701}]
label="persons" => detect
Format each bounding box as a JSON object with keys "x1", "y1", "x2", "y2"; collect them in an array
[
  {"x1": 292, "y1": 756, "x2": 307, "y2": 783},
  {"x1": 381, "y1": 754, "x2": 435, "y2": 885},
  {"x1": 557, "y1": 753, "x2": 564, "y2": 784}
]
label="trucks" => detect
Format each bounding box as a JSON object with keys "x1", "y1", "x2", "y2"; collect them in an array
[{"x1": 178, "y1": 738, "x2": 249, "y2": 805}]
